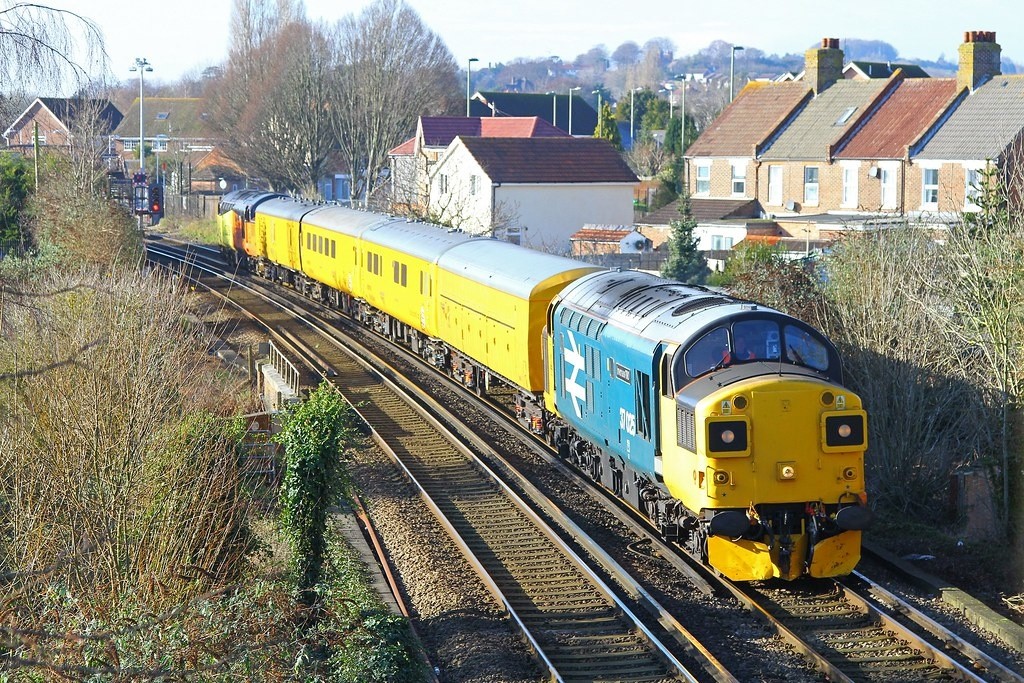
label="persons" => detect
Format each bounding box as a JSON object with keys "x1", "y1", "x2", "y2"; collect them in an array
[{"x1": 722, "y1": 335, "x2": 755, "y2": 364}]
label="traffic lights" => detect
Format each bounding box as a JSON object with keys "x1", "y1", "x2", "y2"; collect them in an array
[{"x1": 148, "y1": 184, "x2": 164, "y2": 215}]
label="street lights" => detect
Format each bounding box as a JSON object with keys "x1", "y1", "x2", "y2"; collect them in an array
[
  {"x1": 730, "y1": 46, "x2": 746, "y2": 103},
  {"x1": 546, "y1": 90, "x2": 557, "y2": 127},
  {"x1": 631, "y1": 86, "x2": 644, "y2": 144},
  {"x1": 569, "y1": 87, "x2": 581, "y2": 136},
  {"x1": 673, "y1": 73, "x2": 687, "y2": 157},
  {"x1": 128, "y1": 56, "x2": 154, "y2": 174},
  {"x1": 466, "y1": 57, "x2": 480, "y2": 117},
  {"x1": 659, "y1": 87, "x2": 673, "y2": 120},
  {"x1": 591, "y1": 90, "x2": 605, "y2": 139}
]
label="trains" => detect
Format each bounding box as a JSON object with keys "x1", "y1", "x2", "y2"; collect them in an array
[{"x1": 217, "y1": 186, "x2": 865, "y2": 585}]
